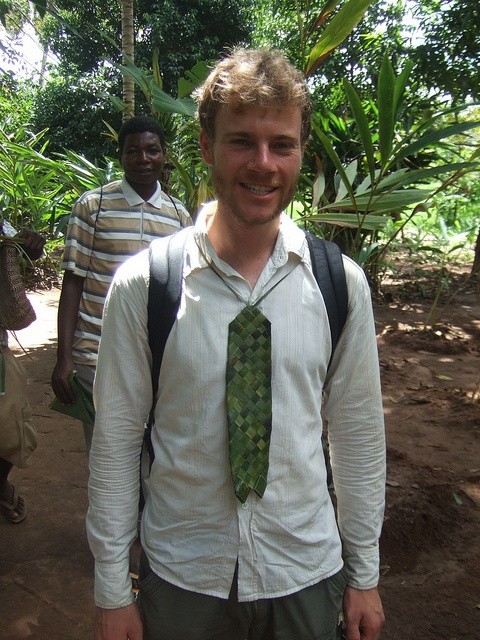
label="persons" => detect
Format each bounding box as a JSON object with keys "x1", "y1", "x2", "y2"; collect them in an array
[
  {"x1": 51, "y1": 115, "x2": 193, "y2": 453},
  {"x1": 85, "y1": 45, "x2": 386, "y2": 640},
  {"x1": 1, "y1": 218, "x2": 46, "y2": 524}
]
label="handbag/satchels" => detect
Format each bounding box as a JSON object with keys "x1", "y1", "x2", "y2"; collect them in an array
[{"x1": 0, "y1": 241, "x2": 36, "y2": 331}]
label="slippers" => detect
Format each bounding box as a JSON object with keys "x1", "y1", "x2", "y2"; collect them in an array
[{"x1": 0, "y1": 484, "x2": 27, "y2": 523}]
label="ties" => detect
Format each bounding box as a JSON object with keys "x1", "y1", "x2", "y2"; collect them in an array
[{"x1": 226, "y1": 305, "x2": 272, "y2": 505}]
